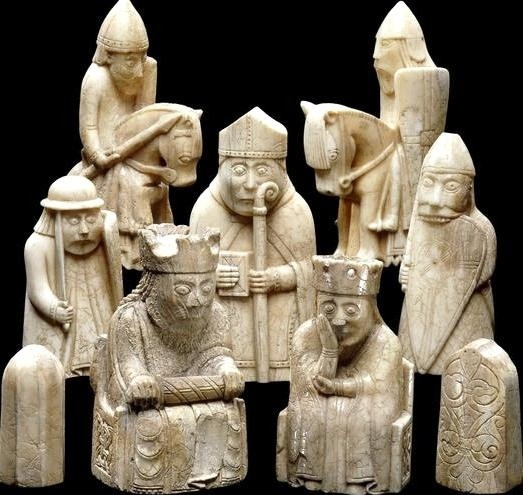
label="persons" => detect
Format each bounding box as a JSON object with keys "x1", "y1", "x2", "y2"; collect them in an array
[
  {"x1": 369, "y1": 0, "x2": 438, "y2": 268},
  {"x1": 77, "y1": 0, "x2": 161, "y2": 273},
  {"x1": 105, "y1": 221, "x2": 245, "y2": 494},
  {"x1": 283, "y1": 253, "x2": 406, "y2": 495},
  {"x1": 187, "y1": 105, "x2": 317, "y2": 388},
  {"x1": 21, "y1": 174, "x2": 121, "y2": 384}
]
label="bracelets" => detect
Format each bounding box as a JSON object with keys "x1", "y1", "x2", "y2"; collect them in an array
[
  {"x1": 331, "y1": 379, "x2": 344, "y2": 397},
  {"x1": 318, "y1": 348, "x2": 338, "y2": 359}
]
